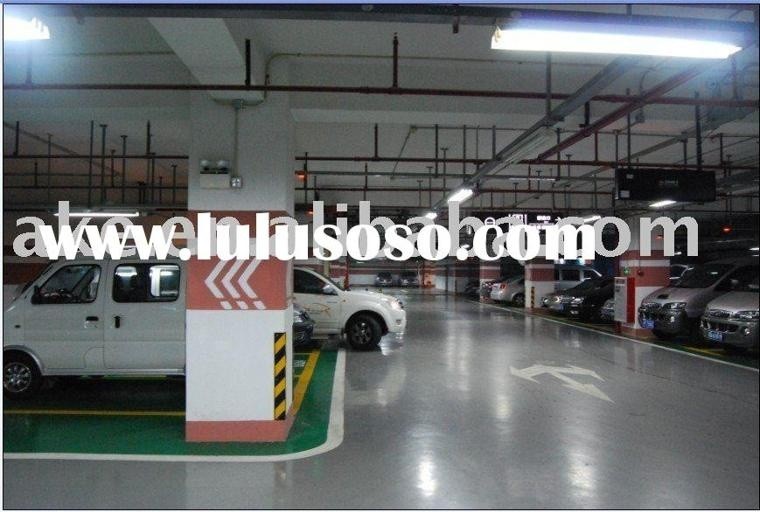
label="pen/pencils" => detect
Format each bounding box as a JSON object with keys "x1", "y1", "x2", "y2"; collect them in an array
[
  {"x1": 4, "y1": 251, "x2": 186, "y2": 403},
  {"x1": 635, "y1": 253, "x2": 759, "y2": 357}
]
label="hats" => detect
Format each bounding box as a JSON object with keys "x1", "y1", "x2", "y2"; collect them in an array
[
  {"x1": 465, "y1": 263, "x2": 622, "y2": 326},
  {"x1": 293, "y1": 266, "x2": 408, "y2": 352},
  {"x1": 373, "y1": 268, "x2": 422, "y2": 288}
]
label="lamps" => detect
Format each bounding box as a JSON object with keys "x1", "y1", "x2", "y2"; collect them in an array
[{"x1": 111, "y1": 274, "x2": 145, "y2": 300}]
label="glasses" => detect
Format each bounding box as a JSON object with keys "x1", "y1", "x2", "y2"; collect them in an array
[
  {"x1": 488, "y1": 16, "x2": 746, "y2": 62},
  {"x1": 200, "y1": 159, "x2": 232, "y2": 192}
]
